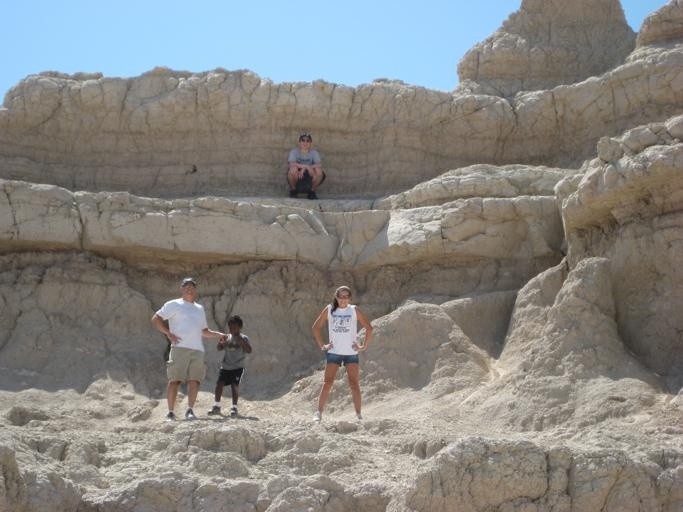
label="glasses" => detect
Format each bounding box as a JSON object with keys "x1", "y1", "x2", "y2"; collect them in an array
[
  {"x1": 301, "y1": 139, "x2": 311, "y2": 143},
  {"x1": 337, "y1": 294, "x2": 350, "y2": 299}
]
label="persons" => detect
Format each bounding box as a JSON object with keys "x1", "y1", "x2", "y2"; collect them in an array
[
  {"x1": 285, "y1": 131, "x2": 327, "y2": 200},
  {"x1": 205, "y1": 315, "x2": 253, "y2": 417},
  {"x1": 149, "y1": 276, "x2": 226, "y2": 422},
  {"x1": 310, "y1": 283, "x2": 371, "y2": 425}
]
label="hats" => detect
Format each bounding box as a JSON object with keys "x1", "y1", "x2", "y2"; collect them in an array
[
  {"x1": 300, "y1": 133, "x2": 311, "y2": 140},
  {"x1": 181, "y1": 278, "x2": 196, "y2": 287}
]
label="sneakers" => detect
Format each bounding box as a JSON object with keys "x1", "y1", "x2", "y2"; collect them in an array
[
  {"x1": 166, "y1": 411, "x2": 175, "y2": 422},
  {"x1": 185, "y1": 408, "x2": 196, "y2": 419},
  {"x1": 290, "y1": 189, "x2": 296, "y2": 198},
  {"x1": 212, "y1": 406, "x2": 220, "y2": 415},
  {"x1": 307, "y1": 188, "x2": 315, "y2": 199},
  {"x1": 230, "y1": 407, "x2": 237, "y2": 416},
  {"x1": 355, "y1": 415, "x2": 363, "y2": 421},
  {"x1": 314, "y1": 411, "x2": 322, "y2": 422}
]
